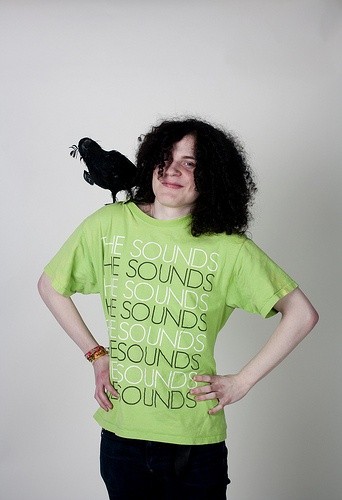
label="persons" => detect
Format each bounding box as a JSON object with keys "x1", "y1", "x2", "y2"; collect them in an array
[{"x1": 35, "y1": 118, "x2": 320, "y2": 500}]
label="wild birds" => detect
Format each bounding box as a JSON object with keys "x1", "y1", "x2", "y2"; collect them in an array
[{"x1": 68, "y1": 137, "x2": 138, "y2": 206}]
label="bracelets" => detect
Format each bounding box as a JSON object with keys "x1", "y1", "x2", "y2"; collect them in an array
[{"x1": 84, "y1": 346, "x2": 108, "y2": 363}]
